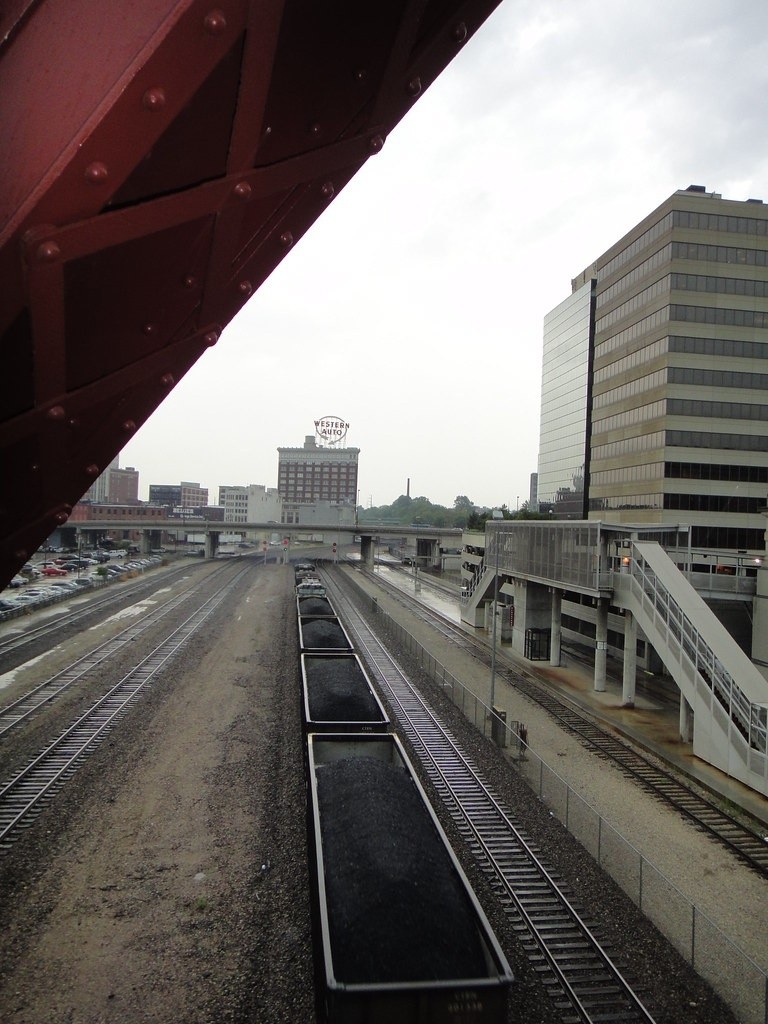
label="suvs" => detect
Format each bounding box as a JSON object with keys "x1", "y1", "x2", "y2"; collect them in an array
[
  {"x1": 128, "y1": 547, "x2": 140, "y2": 556},
  {"x1": 109, "y1": 550, "x2": 127, "y2": 559}
]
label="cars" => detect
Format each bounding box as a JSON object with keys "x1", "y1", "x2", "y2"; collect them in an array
[
  {"x1": 21, "y1": 586, "x2": 57, "y2": 600},
  {"x1": 58, "y1": 555, "x2": 81, "y2": 560},
  {"x1": 8, "y1": 574, "x2": 29, "y2": 588},
  {"x1": 52, "y1": 581, "x2": 78, "y2": 590},
  {"x1": 402, "y1": 558, "x2": 415, "y2": 565},
  {"x1": 36, "y1": 562, "x2": 56, "y2": 569},
  {"x1": 151, "y1": 547, "x2": 166, "y2": 553},
  {"x1": 82, "y1": 551, "x2": 110, "y2": 565},
  {"x1": 0, "y1": 600, "x2": 17, "y2": 610},
  {"x1": 72, "y1": 578, "x2": 92, "y2": 586},
  {"x1": 88, "y1": 573, "x2": 112, "y2": 582},
  {"x1": 49, "y1": 558, "x2": 65, "y2": 565},
  {"x1": 41, "y1": 568, "x2": 67, "y2": 576},
  {"x1": 13, "y1": 596, "x2": 35, "y2": 603},
  {"x1": 89, "y1": 554, "x2": 165, "y2": 575},
  {"x1": 59, "y1": 563, "x2": 78, "y2": 571}
]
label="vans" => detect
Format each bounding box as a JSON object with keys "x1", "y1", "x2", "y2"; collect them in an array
[{"x1": 71, "y1": 560, "x2": 89, "y2": 569}]
